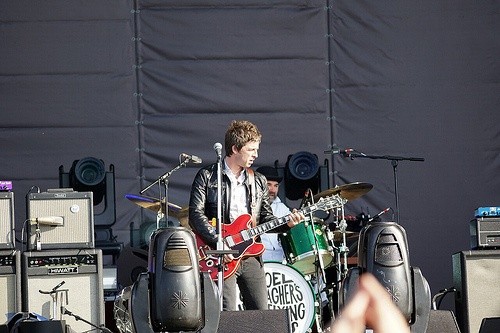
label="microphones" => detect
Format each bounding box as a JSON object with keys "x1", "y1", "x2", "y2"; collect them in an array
[
  {"x1": 29, "y1": 216, "x2": 64, "y2": 225},
  {"x1": 181, "y1": 153, "x2": 202, "y2": 164},
  {"x1": 323, "y1": 148, "x2": 355, "y2": 156},
  {"x1": 213, "y1": 142, "x2": 222, "y2": 164}
]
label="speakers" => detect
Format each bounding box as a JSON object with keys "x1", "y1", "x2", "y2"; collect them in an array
[
  {"x1": 217, "y1": 308, "x2": 292, "y2": 333},
  {"x1": 451, "y1": 250, "x2": 500, "y2": 333},
  {"x1": 0, "y1": 319, "x2": 75, "y2": 333},
  {"x1": 23, "y1": 248, "x2": 106, "y2": 333},
  {"x1": 426, "y1": 310, "x2": 461, "y2": 333},
  {"x1": 0, "y1": 250, "x2": 22, "y2": 325},
  {"x1": 27, "y1": 193, "x2": 95, "y2": 249},
  {"x1": 0, "y1": 192, "x2": 16, "y2": 249}
]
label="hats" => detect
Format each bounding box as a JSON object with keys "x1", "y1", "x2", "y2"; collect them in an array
[{"x1": 256, "y1": 166, "x2": 283, "y2": 182}]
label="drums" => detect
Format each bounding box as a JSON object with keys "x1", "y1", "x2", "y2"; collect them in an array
[{"x1": 235, "y1": 216, "x2": 330, "y2": 332}]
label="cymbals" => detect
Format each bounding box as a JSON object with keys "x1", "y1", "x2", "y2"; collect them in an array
[
  {"x1": 305, "y1": 181, "x2": 372, "y2": 205},
  {"x1": 124, "y1": 193, "x2": 182, "y2": 219}
]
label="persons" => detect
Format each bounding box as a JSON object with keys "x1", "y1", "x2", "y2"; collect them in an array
[
  {"x1": 330, "y1": 272, "x2": 410, "y2": 333},
  {"x1": 256, "y1": 166, "x2": 292, "y2": 265},
  {"x1": 189, "y1": 120, "x2": 306, "y2": 312}
]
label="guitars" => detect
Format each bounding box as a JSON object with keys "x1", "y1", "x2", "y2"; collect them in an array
[{"x1": 194, "y1": 193, "x2": 349, "y2": 282}]
